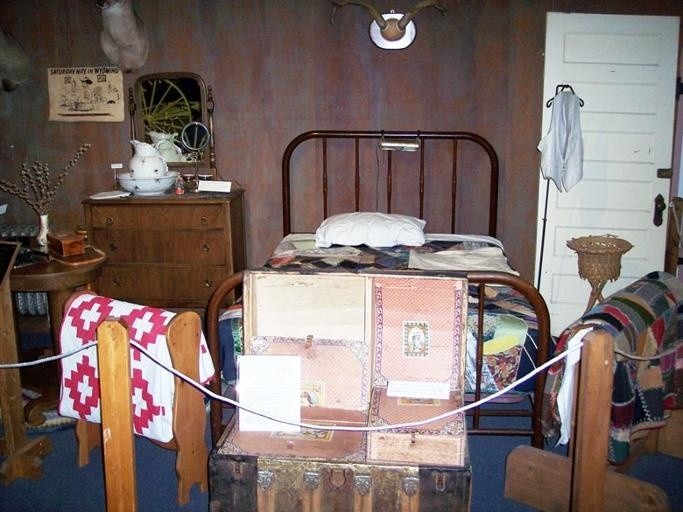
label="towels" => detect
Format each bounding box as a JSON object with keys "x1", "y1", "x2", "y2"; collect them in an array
[{"x1": 56, "y1": 294, "x2": 218, "y2": 443}]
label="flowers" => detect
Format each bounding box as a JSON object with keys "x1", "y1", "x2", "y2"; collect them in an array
[{"x1": 6, "y1": 140, "x2": 94, "y2": 213}]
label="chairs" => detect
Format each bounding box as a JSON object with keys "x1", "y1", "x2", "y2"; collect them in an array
[{"x1": 565, "y1": 262, "x2": 682, "y2": 512}]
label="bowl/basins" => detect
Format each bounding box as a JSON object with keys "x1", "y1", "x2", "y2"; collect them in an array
[{"x1": 117, "y1": 170, "x2": 178, "y2": 197}]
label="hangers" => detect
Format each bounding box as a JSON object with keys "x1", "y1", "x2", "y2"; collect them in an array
[{"x1": 547, "y1": 84, "x2": 585, "y2": 110}]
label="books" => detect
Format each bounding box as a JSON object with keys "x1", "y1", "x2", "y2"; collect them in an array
[{"x1": 88, "y1": 189, "x2": 131, "y2": 200}]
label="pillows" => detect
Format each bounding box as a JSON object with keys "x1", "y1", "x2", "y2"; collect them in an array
[{"x1": 311, "y1": 209, "x2": 427, "y2": 248}]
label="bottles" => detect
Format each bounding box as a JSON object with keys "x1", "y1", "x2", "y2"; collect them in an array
[
  {"x1": 182, "y1": 173, "x2": 195, "y2": 189},
  {"x1": 175, "y1": 178, "x2": 185, "y2": 195},
  {"x1": 197, "y1": 174, "x2": 213, "y2": 186}
]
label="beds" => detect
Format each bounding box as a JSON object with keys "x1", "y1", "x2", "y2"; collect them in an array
[{"x1": 203, "y1": 128, "x2": 568, "y2": 462}]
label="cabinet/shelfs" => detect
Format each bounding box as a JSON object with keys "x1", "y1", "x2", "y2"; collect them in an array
[
  {"x1": 82, "y1": 189, "x2": 246, "y2": 321},
  {"x1": 10, "y1": 247, "x2": 108, "y2": 383}
]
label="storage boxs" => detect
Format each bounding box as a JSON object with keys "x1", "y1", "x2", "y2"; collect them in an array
[{"x1": 202, "y1": 271, "x2": 473, "y2": 510}]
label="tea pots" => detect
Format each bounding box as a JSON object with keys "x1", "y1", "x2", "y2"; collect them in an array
[
  {"x1": 144, "y1": 131, "x2": 183, "y2": 162},
  {"x1": 127, "y1": 140, "x2": 169, "y2": 179}
]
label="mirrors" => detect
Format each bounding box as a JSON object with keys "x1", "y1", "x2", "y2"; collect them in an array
[{"x1": 132, "y1": 72, "x2": 209, "y2": 170}]
